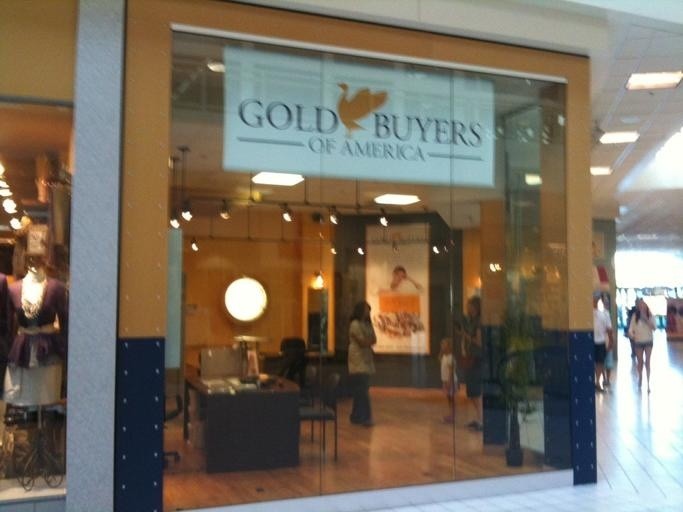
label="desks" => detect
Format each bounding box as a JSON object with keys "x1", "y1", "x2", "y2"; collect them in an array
[{"x1": 184, "y1": 374, "x2": 300, "y2": 474}]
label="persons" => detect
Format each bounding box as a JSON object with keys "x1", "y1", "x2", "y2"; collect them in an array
[
  {"x1": 346, "y1": 301, "x2": 377, "y2": 427},
  {"x1": 592, "y1": 290, "x2": 614, "y2": 394},
  {"x1": 277, "y1": 336, "x2": 318, "y2": 405},
  {"x1": 628, "y1": 297, "x2": 657, "y2": 394},
  {"x1": 624, "y1": 296, "x2": 640, "y2": 358},
  {"x1": 390, "y1": 265, "x2": 417, "y2": 291},
  {"x1": 2, "y1": 257, "x2": 68, "y2": 406},
  {"x1": 437, "y1": 336, "x2": 461, "y2": 424},
  {"x1": 602, "y1": 308, "x2": 614, "y2": 387},
  {"x1": 462, "y1": 294, "x2": 484, "y2": 432}
]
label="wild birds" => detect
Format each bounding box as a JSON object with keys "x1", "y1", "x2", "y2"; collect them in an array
[{"x1": 337, "y1": 81, "x2": 388, "y2": 139}]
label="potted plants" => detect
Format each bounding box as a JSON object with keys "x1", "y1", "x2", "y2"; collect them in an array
[{"x1": 489, "y1": 277, "x2": 549, "y2": 467}]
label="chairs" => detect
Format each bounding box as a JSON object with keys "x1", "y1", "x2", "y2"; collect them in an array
[{"x1": 299, "y1": 365, "x2": 340, "y2": 462}]
